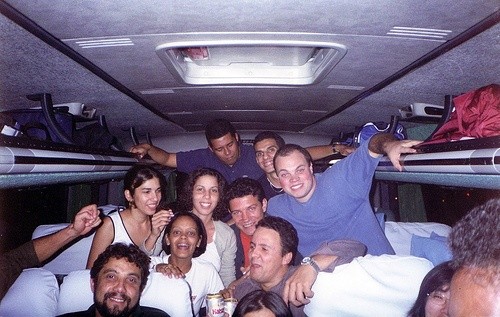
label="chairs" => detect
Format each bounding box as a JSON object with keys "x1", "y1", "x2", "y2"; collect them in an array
[
  {"x1": 31, "y1": 204, "x2": 128, "y2": 286},
  {"x1": 371, "y1": 206, "x2": 455, "y2": 269}
]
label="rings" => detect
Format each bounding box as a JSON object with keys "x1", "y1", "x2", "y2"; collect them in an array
[{"x1": 165, "y1": 267, "x2": 169, "y2": 271}]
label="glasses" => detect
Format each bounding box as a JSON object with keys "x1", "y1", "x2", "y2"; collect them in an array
[{"x1": 427, "y1": 292, "x2": 450, "y2": 304}]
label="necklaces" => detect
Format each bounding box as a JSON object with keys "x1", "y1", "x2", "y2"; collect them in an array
[{"x1": 270, "y1": 179, "x2": 284, "y2": 194}]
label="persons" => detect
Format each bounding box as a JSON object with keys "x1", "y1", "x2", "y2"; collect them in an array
[
  {"x1": 448, "y1": 198, "x2": 500, "y2": 317},
  {"x1": 406, "y1": 260, "x2": 458, "y2": 317},
  {"x1": 84, "y1": 118, "x2": 426, "y2": 317},
  {"x1": 55, "y1": 242, "x2": 169, "y2": 317},
  {"x1": 0, "y1": 191, "x2": 102, "y2": 302}
]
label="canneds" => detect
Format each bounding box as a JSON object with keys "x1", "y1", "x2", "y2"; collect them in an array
[{"x1": 205, "y1": 293, "x2": 238, "y2": 317}]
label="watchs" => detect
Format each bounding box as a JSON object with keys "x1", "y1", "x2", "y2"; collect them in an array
[
  {"x1": 332, "y1": 141, "x2": 342, "y2": 154},
  {"x1": 300, "y1": 256, "x2": 320, "y2": 274}
]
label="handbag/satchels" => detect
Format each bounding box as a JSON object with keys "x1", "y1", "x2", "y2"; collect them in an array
[
  {"x1": 72, "y1": 122, "x2": 118, "y2": 153},
  {"x1": 410, "y1": 83, "x2": 500, "y2": 148},
  {"x1": 0, "y1": 106, "x2": 75, "y2": 145},
  {"x1": 331, "y1": 121, "x2": 408, "y2": 160}
]
